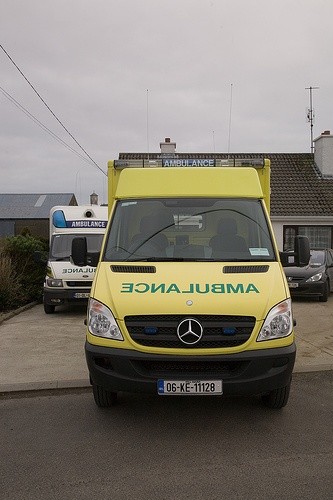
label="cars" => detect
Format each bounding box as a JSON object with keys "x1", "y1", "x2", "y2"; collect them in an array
[{"x1": 282, "y1": 245, "x2": 333, "y2": 302}]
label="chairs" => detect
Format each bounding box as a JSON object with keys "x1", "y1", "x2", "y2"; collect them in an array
[
  {"x1": 207, "y1": 218, "x2": 250, "y2": 257},
  {"x1": 127, "y1": 215, "x2": 168, "y2": 258}
]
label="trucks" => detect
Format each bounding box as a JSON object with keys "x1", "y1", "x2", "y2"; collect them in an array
[
  {"x1": 71, "y1": 156, "x2": 310, "y2": 407},
  {"x1": 37, "y1": 204, "x2": 110, "y2": 313}
]
